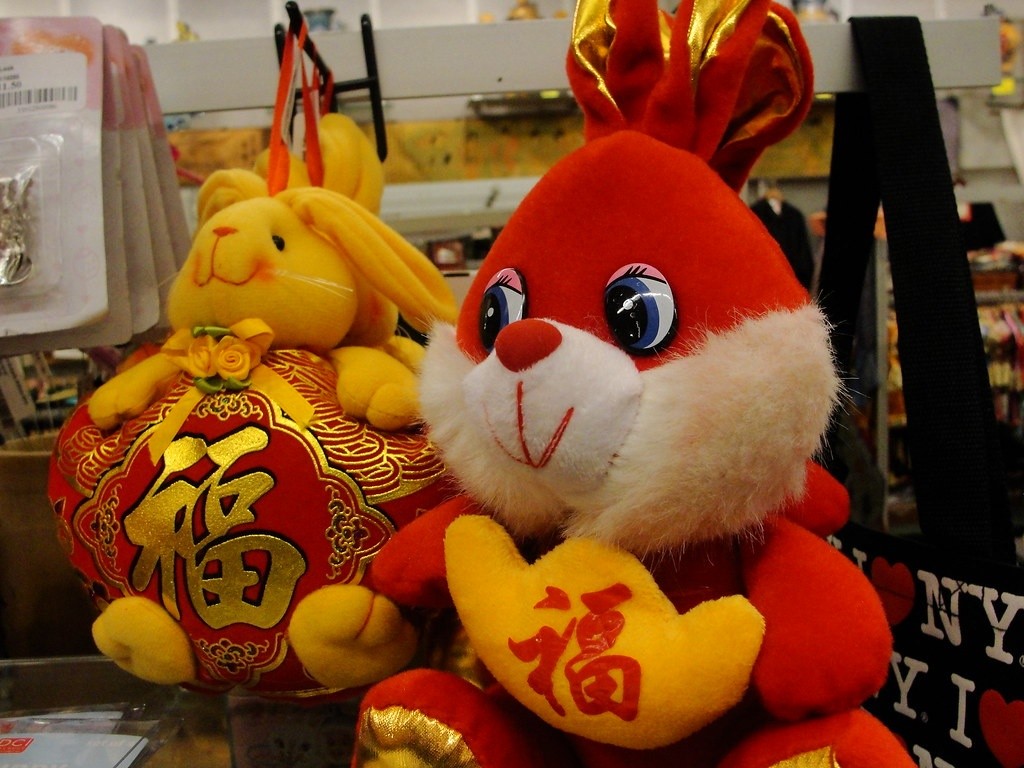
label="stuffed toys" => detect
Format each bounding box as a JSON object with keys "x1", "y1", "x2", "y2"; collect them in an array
[
  {"x1": 50, "y1": 115, "x2": 458, "y2": 701},
  {"x1": 354, "y1": 0, "x2": 920, "y2": 768}
]
